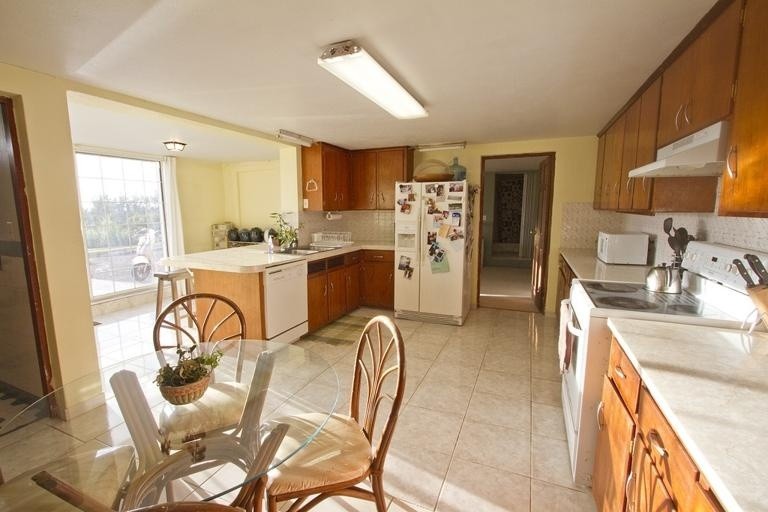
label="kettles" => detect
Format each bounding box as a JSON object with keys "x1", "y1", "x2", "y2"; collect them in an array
[{"x1": 645, "y1": 263, "x2": 682, "y2": 294}]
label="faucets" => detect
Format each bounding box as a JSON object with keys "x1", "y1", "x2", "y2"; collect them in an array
[{"x1": 269, "y1": 234, "x2": 274, "y2": 239}]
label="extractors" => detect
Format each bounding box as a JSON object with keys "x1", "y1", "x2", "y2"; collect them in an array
[{"x1": 628, "y1": 121, "x2": 728, "y2": 178}]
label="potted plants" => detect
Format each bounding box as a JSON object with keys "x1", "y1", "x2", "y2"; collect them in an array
[
  {"x1": 153, "y1": 343, "x2": 224, "y2": 404},
  {"x1": 272, "y1": 212, "x2": 304, "y2": 250}
]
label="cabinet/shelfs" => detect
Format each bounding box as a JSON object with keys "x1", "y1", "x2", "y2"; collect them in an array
[
  {"x1": 656, "y1": 0, "x2": 744, "y2": 149},
  {"x1": 594, "y1": 127, "x2": 606, "y2": 212},
  {"x1": 618, "y1": 87, "x2": 641, "y2": 216},
  {"x1": 308, "y1": 251, "x2": 347, "y2": 335},
  {"x1": 589, "y1": 316, "x2": 768, "y2": 512},
  {"x1": 599, "y1": 106, "x2": 625, "y2": 211},
  {"x1": 556, "y1": 248, "x2": 654, "y2": 331},
  {"x1": 345, "y1": 247, "x2": 361, "y2": 315},
  {"x1": 362, "y1": 245, "x2": 395, "y2": 310},
  {"x1": 714, "y1": 0, "x2": 768, "y2": 220},
  {"x1": 352, "y1": 145, "x2": 415, "y2": 211},
  {"x1": 188, "y1": 253, "x2": 308, "y2": 359},
  {"x1": 633, "y1": 74, "x2": 661, "y2": 212},
  {"x1": 301, "y1": 142, "x2": 351, "y2": 212}
]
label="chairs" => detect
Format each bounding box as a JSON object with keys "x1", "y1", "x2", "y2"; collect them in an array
[
  {"x1": 151, "y1": 293, "x2": 250, "y2": 504},
  {"x1": 259, "y1": 315, "x2": 405, "y2": 512}
]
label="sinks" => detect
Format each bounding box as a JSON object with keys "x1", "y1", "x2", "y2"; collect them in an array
[
  {"x1": 277, "y1": 250, "x2": 321, "y2": 255},
  {"x1": 292, "y1": 246, "x2": 341, "y2": 251}
]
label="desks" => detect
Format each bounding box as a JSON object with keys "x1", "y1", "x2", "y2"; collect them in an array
[{"x1": 0, "y1": 339, "x2": 339, "y2": 512}]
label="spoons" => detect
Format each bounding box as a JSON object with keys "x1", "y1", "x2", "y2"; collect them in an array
[{"x1": 664, "y1": 217, "x2": 697, "y2": 266}]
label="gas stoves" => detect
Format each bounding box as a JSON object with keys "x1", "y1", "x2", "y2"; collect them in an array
[{"x1": 572, "y1": 241, "x2": 768, "y2": 332}]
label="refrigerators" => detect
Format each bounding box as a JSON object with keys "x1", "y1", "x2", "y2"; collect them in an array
[{"x1": 393, "y1": 179, "x2": 474, "y2": 327}]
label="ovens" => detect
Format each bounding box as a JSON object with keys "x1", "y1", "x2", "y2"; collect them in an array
[{"x1": 558, "y1": 299, "x2": 612, "y2": 482}]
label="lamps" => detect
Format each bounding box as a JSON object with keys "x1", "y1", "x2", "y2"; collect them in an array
[
  {"x1": 162, "y1": 141, "x2": 186, "y2": 153},
  {"x1": 318, "y1": 39, "x2": 429, "y2": 120}
]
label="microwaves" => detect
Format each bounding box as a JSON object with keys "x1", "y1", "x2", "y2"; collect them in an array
[{"x1": 596, "y1": 230, "x2": 649, "y2": 265}]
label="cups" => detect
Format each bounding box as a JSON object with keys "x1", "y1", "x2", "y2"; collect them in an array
[{"x1": 313, "y1": 231, "x2": 352, "y2": 245}]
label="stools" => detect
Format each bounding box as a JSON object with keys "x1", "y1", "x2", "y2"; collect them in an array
[{"x1": 154, "y1": 268, "x2": 193, "y2": 348}]
